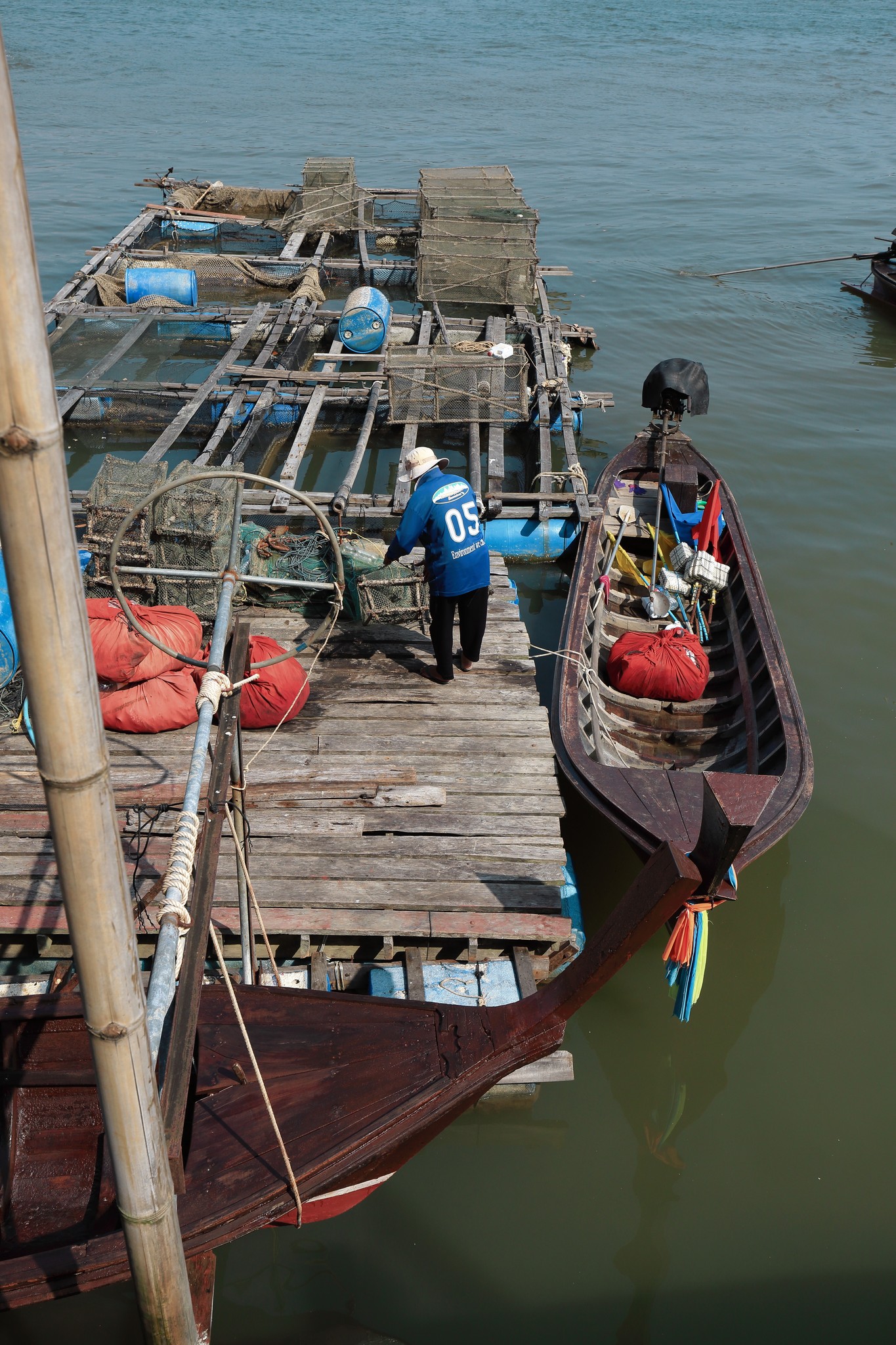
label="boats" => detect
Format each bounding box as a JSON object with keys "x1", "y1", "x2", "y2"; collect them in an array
[
  {"x1": 550, "y1": 357, "x2": 814, "y2": 902},
  {"x1": 0, "y1": 843, "x2": 701, "y2": 1311},
  {"x1": 870, "y1": 247, "x2": 896, "y2": 303}
]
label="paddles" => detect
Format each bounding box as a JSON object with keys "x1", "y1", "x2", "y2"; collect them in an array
[
  {"x1": 680, "y1": 252, "x2": 896, "y2": 279},
  {"x1": 591, "y1": 505, "x2": 642, "y2": 611}
]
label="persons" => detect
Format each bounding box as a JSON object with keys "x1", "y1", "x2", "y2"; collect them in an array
[{"x1": 382, "y1": 445, "x2": 490, "y2": 684}]
